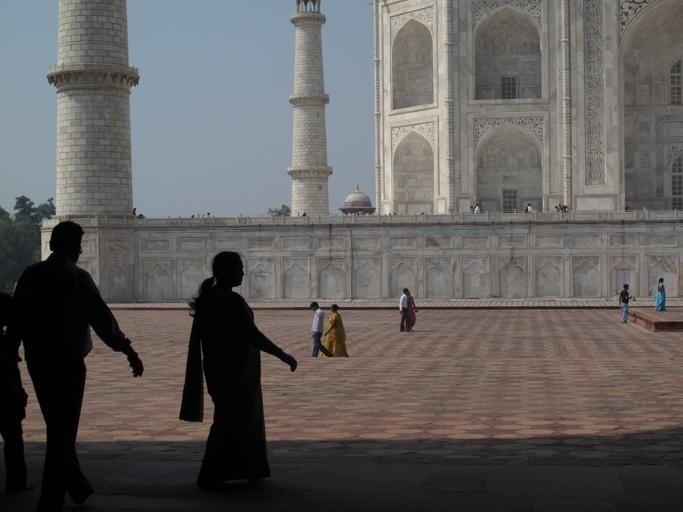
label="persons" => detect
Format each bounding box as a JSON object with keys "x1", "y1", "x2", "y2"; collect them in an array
[
  {"x1": 407, "y1": 291, "x2": 417, "y2": 332},
  {"x1": 469, "y1": 202, "x2": 569, "y2": 213},
  {"x1": 187, "y1": 250, "x2": 298, "y2": 489},
  {"x1": 318, "y1": 304, "x2": 350, "y2": 357},
  {"x1": 655, "y1": 278, "x2": 666, "y2": 311},
  {"x1": 0, "y1": 222, "x2": 142, "y2": 511},
  {"x1": 398, "y1": 287, "x2": 410, "y2": 333},
  {"x1": 309, "y1": 301, "x2": 333, "y2": 358},
  {"x1": 618, "y1": 284, "x2": 632, "y2": 324},
  {"x1": 0, "y1": 291, "x2": 35, "y2": 495}
]
